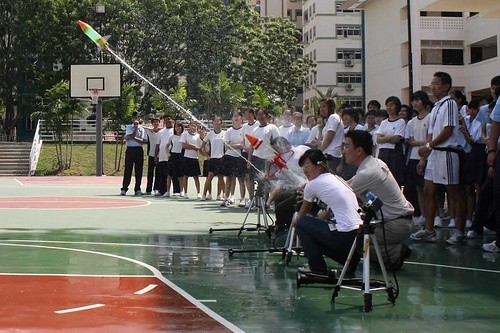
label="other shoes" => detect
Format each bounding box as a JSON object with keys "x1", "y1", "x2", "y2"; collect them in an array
[
  {"x1": 448, "y1": 218, "x2": 455, "y2": 227},
  {"x1": 144, "y1": 191, "x2": 152, "y2": 195},
  {"x1": 413, "y1": 216, "x2": 426, "y2": 226},
  {"x1": 465, "y1": 220, "x2": 472, "y2": 227},
  {"x1": 121, "y1": 190, "x2": 126, "y2": 196},
  {"x1": 135, "y1": 190, "x2": 142, "y2": 195},
  {"x1": 389, "y1": 244, "x2": 410, "y2": 269},
  {"x1": 299, "y1": 265, "x2": 322, "y2": 275}
]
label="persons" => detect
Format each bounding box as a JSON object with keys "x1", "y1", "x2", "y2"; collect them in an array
[
  {"x1": 338, "y1": 91, "x2": 434, "y2": 224},
  {"x1": 298, "y1": 131, "x2": 415, "y2": 269},
  {"x1": 433, "y1": 75, "x2": 500, "y2": 253},
  {"x1": 264, "y1": 98, "x2": 345, "y2": 233},
  {"x1": 241, "y1": 107, "x2": 280, "y2": 211},
  {"x1": 140, "y1": 115, "x2": 202, "y2": 199},
  {"x1": 121, "y1": 111, "x2": 145, "y2": 196},
  {"x1": 197, "y1": 110, "x2": 247, "y2": 208},
  {"x1": 293, "y1": 149, "x2": 362, "y2": 277},
  {"x1": 409, "y1": 72, "x2": 467, "y2": 245}
]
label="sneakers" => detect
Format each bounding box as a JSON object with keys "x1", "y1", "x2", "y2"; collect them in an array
[
  {"x1": 466, "y1": 230, "x2": 483, "y2": 239},
  {"x1": 434, "y1": 216, "x2": 442, "y2": 228},
  {"x1": 155, "y1": 190, "x2": 254, "y2": 206},
  {"x1": 483, "y1": 240, "x2": 500, "y2": 252},
  {"x1": 483, "y1": 227, "x2": 496, "y2": 235},
  {"x1": 446, "y1": 231, "x2": 466, "y2": 244},
  {"x1": 410, "y1": 229, "x2": 437, "y2": 242}
]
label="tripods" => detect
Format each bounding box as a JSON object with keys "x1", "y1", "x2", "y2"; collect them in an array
[
  {"x1": 282, "y1": 205, "x2": 307, "y2": 261},
  {"x1": 237, "y1": 188, "x2": 272, "y2": 239},
  {"x1": 331, "y1": 220, "x2": 396, "y2": 311}
]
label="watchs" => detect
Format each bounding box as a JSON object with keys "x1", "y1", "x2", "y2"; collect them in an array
[{"x1": 486, "y1": 150, "x2": 495, "y2": 154}]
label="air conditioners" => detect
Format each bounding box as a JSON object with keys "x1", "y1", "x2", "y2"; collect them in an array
[
  {"x1": 346, "y1": 59, "x2": 354, "y2": 66},
  {"x1": 346, "y1": 83, "x2": 354, "y2": 90}
]
